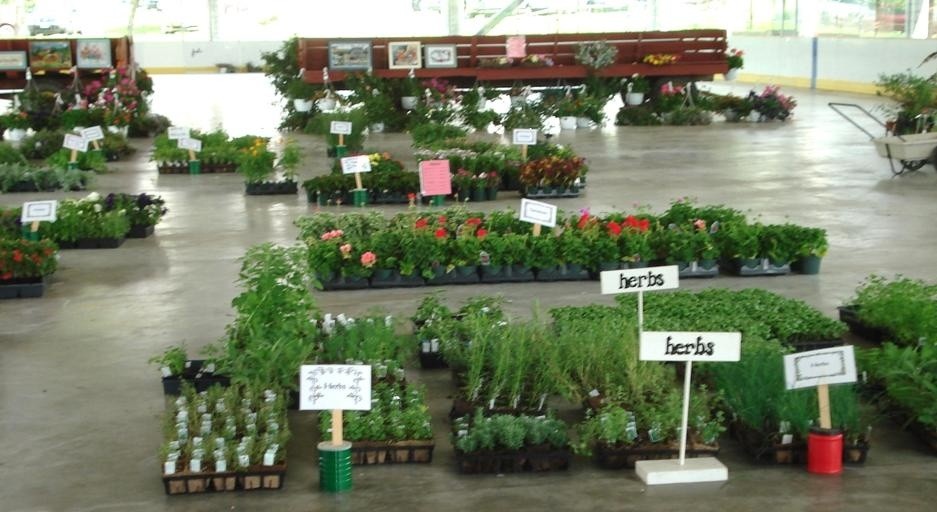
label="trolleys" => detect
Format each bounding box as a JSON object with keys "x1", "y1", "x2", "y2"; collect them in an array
[{"x1": 827, "y1": 99, "x2": 937, "y2": 178}]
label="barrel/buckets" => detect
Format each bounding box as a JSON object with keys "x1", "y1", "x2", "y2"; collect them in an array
[
  {"x1": 317, "y1": 442, "x2": 353, "y2": 490},
  {"x1": 808, "y1": 427, "x2": 843, "y2": 473}
]
